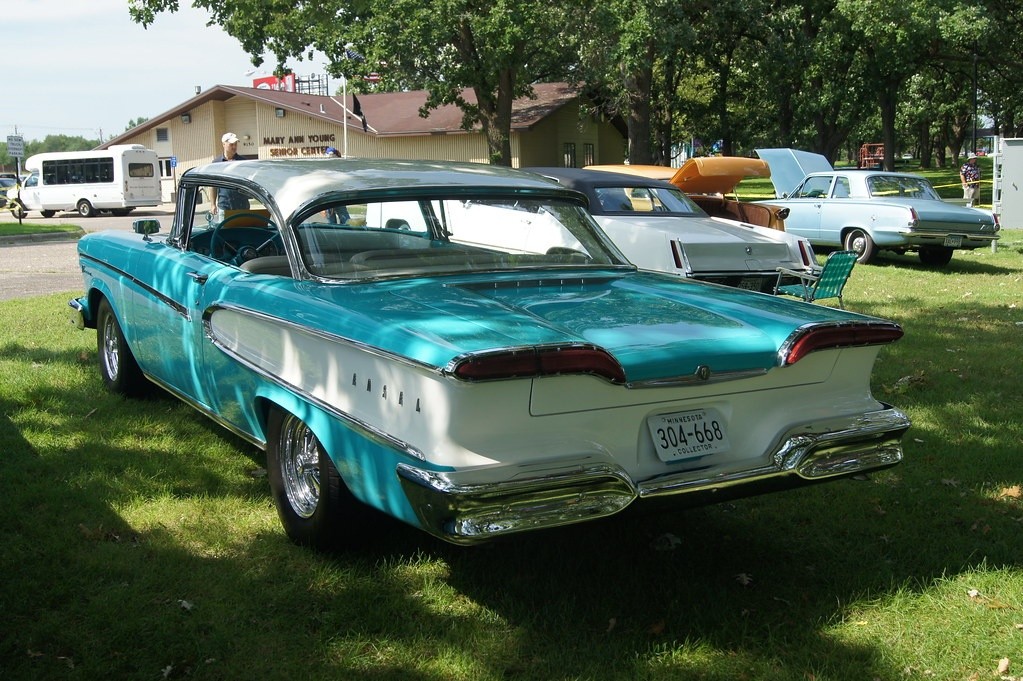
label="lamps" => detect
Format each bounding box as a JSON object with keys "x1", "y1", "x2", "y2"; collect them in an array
[
  {"x1": 182, "y1": 114, "x2": 190, "y2": 124},
  {"x1": 275, "y1": 108, "x2": 285, "y2": 117}
]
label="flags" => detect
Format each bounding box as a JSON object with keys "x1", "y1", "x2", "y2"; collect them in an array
[
  {"x1": 345, "y1": 46, "x2": 388, "y2": 84},
  {"x1": 353, "y1": 92, "x2": 368, "y2": 132}
]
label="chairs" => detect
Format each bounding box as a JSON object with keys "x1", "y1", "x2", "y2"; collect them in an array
[{"x1": 773, "y1": 250, "x2": 860, "y2": 310}]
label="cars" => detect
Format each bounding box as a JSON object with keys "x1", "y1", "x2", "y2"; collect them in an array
[
  {"x1": 68, "y1": 158, "x2": 916, "y2": 557},
  {"x1": 365, "y1": 165, "x2": 822, "y2": 302},
  {"x1": 0, "y1": 173, "x2": 37, "y2": 207},
  {"x1": 744, "y1": 146, "x2": 1000, "y2": 272},
  {"x1": 900, "y1": 141, "x2": 988, "y2": 159},
  {"x1": 578, "y1": 156, "x2": 791, "y2": 234}
]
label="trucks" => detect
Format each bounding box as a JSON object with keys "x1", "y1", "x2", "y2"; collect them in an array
[
  {"x1": 857, "y1": 143, "x2": 886, "y2": 171},
  {"x1": 4, "y1": 144, "x2": 165, "y2": 218}
]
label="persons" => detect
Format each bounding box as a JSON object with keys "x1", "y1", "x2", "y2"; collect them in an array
[
  {"x1": 207, "y1": 132, "x2": 252, "y2": 224},
  {"x1": 959, "y1": 152, "x2": 982, "y2": 207},
  {"x1": 319, "y1": 148, "x2": 350, "y2": 228}
]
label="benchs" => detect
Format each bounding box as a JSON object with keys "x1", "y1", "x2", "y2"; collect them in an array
[{"x1": 239, "y1": 247, "x2": 472, "y2": 274}]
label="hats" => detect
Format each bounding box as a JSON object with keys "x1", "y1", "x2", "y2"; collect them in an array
[
  {"x1": 326, "y1": 147, "x2": 341, "y2": 157},
  {"x1": 222, "y1": 132, "x2": 240, "y2": 144},
  {"x1": 967, "y1": 152, "x2": 977, "y2": 160}
]
